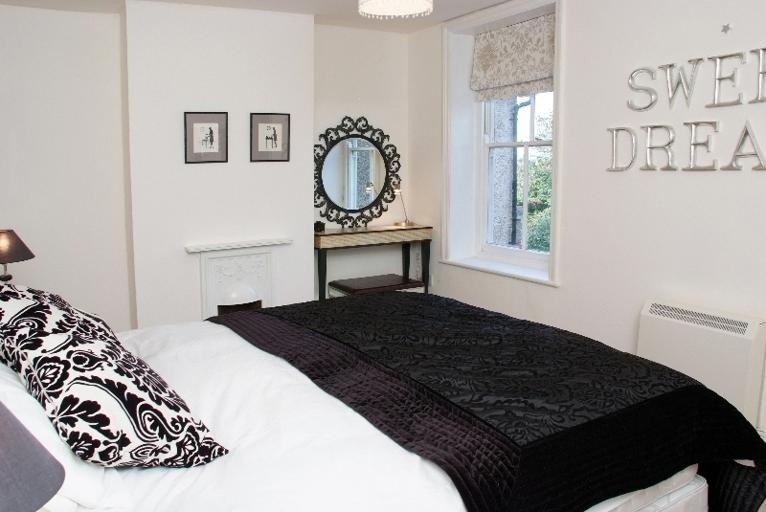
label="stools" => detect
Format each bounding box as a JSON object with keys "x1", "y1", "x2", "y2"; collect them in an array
[{"x1": 327, "y1": 273, "x2": 426, "y2": 296}]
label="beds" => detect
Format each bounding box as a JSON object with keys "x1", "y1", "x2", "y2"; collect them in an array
[{"x1": 0, "y1": 292, "x2": 766, "y2": 510}]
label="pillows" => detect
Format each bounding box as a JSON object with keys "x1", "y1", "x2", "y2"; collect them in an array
[{"x1": 1, "y1": 284, "x2": 229, "y2": 470}]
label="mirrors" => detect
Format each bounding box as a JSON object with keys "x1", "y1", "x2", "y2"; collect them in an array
[{"x1": 318, "y1": 133, "x2": 389, "y2": 214}]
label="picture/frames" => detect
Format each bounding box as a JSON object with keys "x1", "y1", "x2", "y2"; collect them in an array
[
  {"x1": 183, "y1": 112, "x2": 229, "y2": 165},
  {"x1": 249, "y1": 112, "x2": 290, "y2": 163}
]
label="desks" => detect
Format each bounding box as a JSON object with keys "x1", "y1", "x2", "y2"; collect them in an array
[{"x1": 314, "y1": 223, "x2": 433, "y2": 300}]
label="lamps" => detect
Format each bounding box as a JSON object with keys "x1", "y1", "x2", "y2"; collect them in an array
[{"x1": 0, "y1": 230, "x2": 35, "y2": 281}]
